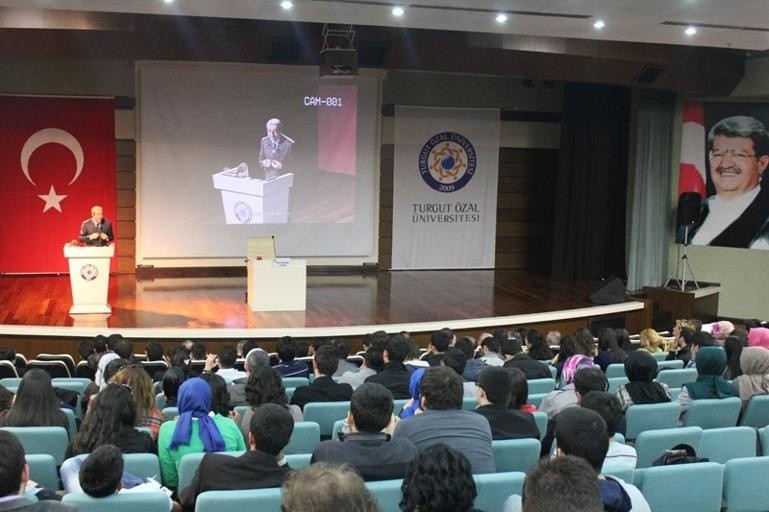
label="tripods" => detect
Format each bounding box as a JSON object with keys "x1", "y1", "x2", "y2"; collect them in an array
[{"x1": 663, "y1": 226, "x2": 700, "y2": 292}]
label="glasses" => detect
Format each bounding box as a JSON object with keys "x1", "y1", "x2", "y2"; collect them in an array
[{"x1": 709, "y1": 149, "x2": 757, "y2": 161}]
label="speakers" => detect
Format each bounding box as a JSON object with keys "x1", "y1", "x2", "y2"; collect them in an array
[
  {"x1": 587, "y1": 274, "x2": 626, "y2": 305},
  {"x1": 381, "y1": 104, "x2": 394, "y2": 116},
  {"x1": 678, "y1": 192, "x2": 702, "y2": 225},
  {"x1": 319, "y1": 49, "x2": 359, "y2": 80}
]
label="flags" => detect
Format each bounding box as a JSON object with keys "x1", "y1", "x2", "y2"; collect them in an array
[
  {"x1": 1, "y1": 97, "x2": 119, "y2": 276},
  {"x1": 681, "y1": 104, "x2": 708, "y2": 198}
]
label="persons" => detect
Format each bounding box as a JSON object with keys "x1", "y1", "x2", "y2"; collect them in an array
[
  {"x1": 259, "y1": 117, "x2": 296, "y2": 180},
  {"x1": 678, "y1": 115, "x2": 768, "y2": 249},
  {"x1": 79, "y1": 206, "x2": 114, "y2": 246},
  {"x1": 1, "y1": 317, "x2": 769, "y2": 512}
]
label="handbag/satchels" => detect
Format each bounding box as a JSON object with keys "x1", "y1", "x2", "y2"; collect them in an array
[{"x1": 652, "y1": 444, "x2": 710, "y2": 467}]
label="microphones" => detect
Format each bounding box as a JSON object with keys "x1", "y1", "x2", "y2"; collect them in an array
[{"x1": 272, "y1": 235, "x2": 276, "y2": 258}]
